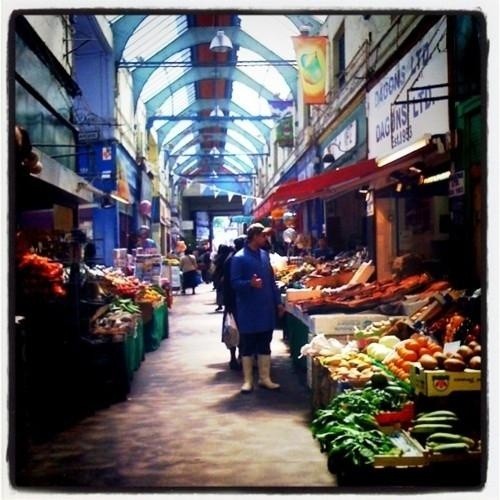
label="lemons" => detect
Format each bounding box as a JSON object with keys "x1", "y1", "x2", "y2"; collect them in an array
[{"x1": 142, "y1": 290, "x2": 161, "y2": 301}]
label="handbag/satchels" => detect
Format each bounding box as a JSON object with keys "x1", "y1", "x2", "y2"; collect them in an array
[{"x1": 191, "y1": 272, "x2": 203, "y2": 284}]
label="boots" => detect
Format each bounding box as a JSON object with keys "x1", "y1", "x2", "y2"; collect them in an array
[
  {"x1": 240, "y1": 353, "x2": 254, "y2": 392},
  {"x1": 256, "y1": 354, "x2": 280, "y2": 389}
]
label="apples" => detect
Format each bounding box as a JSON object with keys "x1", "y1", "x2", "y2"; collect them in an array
[{"x1": 464, "y1": 325, "x2": 481, "y2": 344}]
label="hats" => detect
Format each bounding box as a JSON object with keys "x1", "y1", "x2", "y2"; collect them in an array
[{"x1": 246, "y1": 222, "x2": 274, "y2": 237}]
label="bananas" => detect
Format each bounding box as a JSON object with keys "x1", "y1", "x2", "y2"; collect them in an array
[{"x1": 353, "y1": 319, "x2": 390, "y2": 337}]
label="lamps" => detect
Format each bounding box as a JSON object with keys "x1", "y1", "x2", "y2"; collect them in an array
[
  {"x1": 78, "y1": 175, "x2": 116, "y2": 208},
  {"x1": 322, "y1": 142, "x2": 358, "y2": 163},
  {"x1": 357, "y1": 158, "x2": 457, "y2": 195}
]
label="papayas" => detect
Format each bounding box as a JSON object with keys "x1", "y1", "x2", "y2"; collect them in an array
[{"x1": 420, "y1": 341, "x2": 482, "y2": 372}]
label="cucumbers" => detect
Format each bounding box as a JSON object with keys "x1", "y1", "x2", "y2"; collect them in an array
[{"x1": 408, "y1": 407, "x2": 476, "y2": 454}]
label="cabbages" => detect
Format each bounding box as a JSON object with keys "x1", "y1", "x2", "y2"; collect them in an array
[{"x1": 367, "y1": 335, "x2": 401, "y2": 362}]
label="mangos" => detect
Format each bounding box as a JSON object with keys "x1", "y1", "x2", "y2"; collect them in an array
[{"x1": 321, "y1": 352, "x2": 382, "y2": 382}]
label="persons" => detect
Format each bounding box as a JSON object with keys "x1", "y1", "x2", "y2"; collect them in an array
[
  {"x1": 196, "y1": 240, "x2": 213, "y2": 286},
  {"x1": 221, "y1": 235, "x2": 259, "y2": 371},
  {"x1": 126, "y1": 235, "x2": 144, "y2": 254},
  {"x1": 230, "y1": 221, "x2": 285, "y2": 392},
  {"x1": 137, "y1": 223, "x2": 156, "y2": 248},
  {"x1": 179, "y1": 248, "x2": 197, "y2": 294},
  {"x1": 314, "y1": 237, "x2": 334, "y2": 259},
  {"x1": 211, "y1": 244, "x2": 233, "y2": 311}
]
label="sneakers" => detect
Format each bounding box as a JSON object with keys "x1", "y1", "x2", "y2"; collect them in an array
[{"x1": 230, "y1": 360, "x2": 239, "y2": 370}]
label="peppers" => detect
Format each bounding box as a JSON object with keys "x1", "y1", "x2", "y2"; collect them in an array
[{"x1": 109, "y1": 294, "x2": 142, "y2": 313}]
